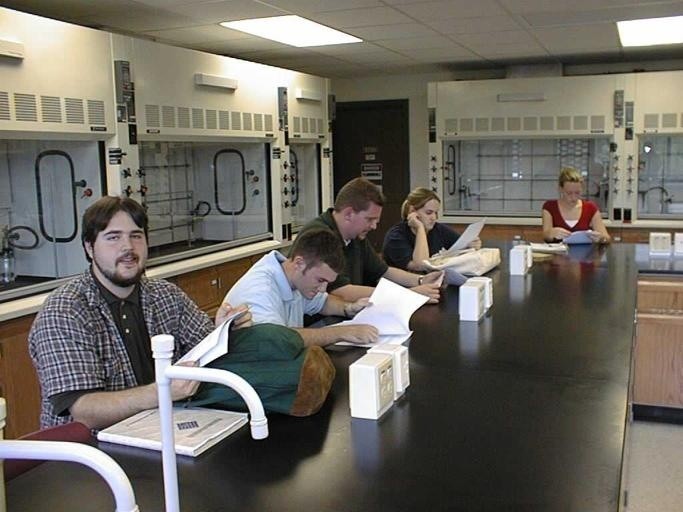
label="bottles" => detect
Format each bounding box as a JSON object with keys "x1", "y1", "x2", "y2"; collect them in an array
[
  {"x1": 511, "y1": 235, "x2": 524, "y2": 245},
  {"x1": 0, "y1": 247, "x2": 16, "y2": 286}
]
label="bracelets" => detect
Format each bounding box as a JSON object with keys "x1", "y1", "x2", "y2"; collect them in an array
[
  {"x1": 342, "y1": 302, "x2": 348, "y2": 318},
  {"x1": 418, "y1": 276, "x2": 426, "y2": 287},
  {"x1": 601, "y1": 238, "x2": 607, "y2": 244}
]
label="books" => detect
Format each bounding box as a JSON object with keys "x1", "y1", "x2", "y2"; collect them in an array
[
  {"x1": 100, "y1": 404, "x2": 253, "y2": 458},
  {"x1": 530, "y1": 240, "x2": 570, "y2": 255},
  {"x1": 321, "y1": 277, "x2": 432, "y2": 350},
  {"x1": 172, "y1": 306, "x2": 251, "y2": 367},
  {"x1": 563, "y1": 230, "x2": 595, "y2": 244}
]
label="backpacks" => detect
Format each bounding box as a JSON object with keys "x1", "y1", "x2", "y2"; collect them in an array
[{"x1": 187, "y1": 323, "x2": 335, "y2": 417}]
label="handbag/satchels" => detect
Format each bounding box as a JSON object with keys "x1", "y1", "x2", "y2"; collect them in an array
[{"x1": 422, "y1": 248, "x2": 501, "y2": 276}]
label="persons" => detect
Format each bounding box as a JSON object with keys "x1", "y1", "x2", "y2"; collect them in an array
[
  {"x1": 217, "y1": 226, "x2": 380, "y2": 351},
  {"x1": 381, "y1": 184, "x2": 486, "y2": 265},
  {"x1": 26, "y1": 194, "x2": 253, "y2": 431},
  {"x1": 285, "y1": 179, "x2": 442, "y2": 307},
  {"x1": 541, "y1": 167, "x2": 610, "y2": 246}
]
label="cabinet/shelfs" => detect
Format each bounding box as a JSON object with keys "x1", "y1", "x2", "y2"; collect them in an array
[
  {"x1": 442, "y1": 226, "x2": 683, "y2": 243},
  {"x1": 0, "y1": 246, "x2": 292, "y2": 440}
]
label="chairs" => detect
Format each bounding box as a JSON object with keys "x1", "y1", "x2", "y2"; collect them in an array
[{"x1": 2, "y1": 420, "x2": 92, "y2": 482}]
